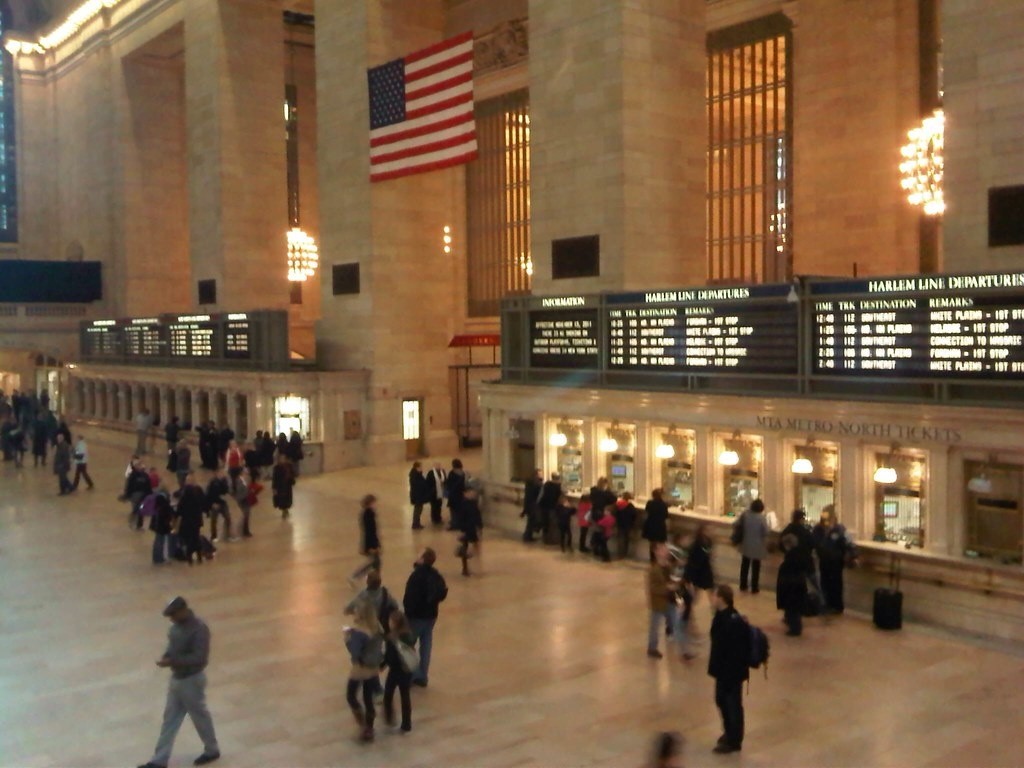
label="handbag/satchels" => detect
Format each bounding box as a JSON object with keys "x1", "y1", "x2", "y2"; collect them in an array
[
  {"x1": 73, "y1": 453, "x2": 84, "y2": 459},
  {"x1": 395, "y1": 641, "x2": 420, "y2": 674},
  {"x1": 731, "y1": 513, "x2": 745, "y2": 544}
]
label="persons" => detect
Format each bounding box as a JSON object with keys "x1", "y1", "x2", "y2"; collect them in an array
[
  {"x1": 451, "y1": 488, "x2": 485, "y2": 577},
  {"x1": 138, "y1": 596, "x2": 220, "y2": 768},
  {"x1": 343, "y1": 570, "x2": 416, "y2": 741},
  {"x1": 446, "y1": 458, "x2": 466, "y2": 524},
  {"x1": 646, "y1": 523, "x2": 714, "y2": 661},
  {"x1": 0, "y1": 388, "x2": 71, "y2": 466},
  {"x1": 731, "y1": 498, "x2": 768, "y2": 593},
  {"x1": 53, "y1": 433, "x2": 94, "y2": 495},
  {"x1": 520, "y1": 469, "x2": 575, "y2": 552},
  {"x1": 641, "y1": 487, "x2": 669, "y2": 561},
  {"x1": 645, "y1": 731, "x2": 685, "y2": 768},
  {"x1": 123, "y1": 455, "x2": 254, "y2": 564},
  {"x1": 409, "y1": 460, "x2": 446, "y2": 531},
  {"x1": 346, "y1": 494, "x2": 382, "y2": 587},
  {"x1": 708, "y1": 584, "x2": 751, "y2": 753},
  {"x1": 778, "y1": 505, "x2": 847, "y2": 638},
  {"x1": 579, "y1": 477, "x2": 636, "y2": 563},
  {"x1": 402, "y1": 547, "x2": 449, "y2": 686},
  {"x1": 133, "y1": 408, "x2": 304, "y2": 520}
]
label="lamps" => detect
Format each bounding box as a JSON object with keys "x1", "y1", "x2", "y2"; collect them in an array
[
  {"x1": 598, "y1": 419, "x2": 621, "y2": 453},
  {"x1": 284, "y1": 13, "x2": 319, "y2": 282},
  {"x1": 966, "y1": 455, "x2": 997, "y2": 495},
  {"x1": 896, "y1": 105, "x2": 948, "y2": 217},
  {"x1": 548, "y1": 416, "x2": 569, "y2": 447},
  {"x1": 870, "y1": 438, "x2": 904, "y2": 484},
  {"x1": 717, "y1": 429, "x2": 742, "y2": 466},
  {"x1": 655, "y1": 422, "x2": 679, "y2": 459},
  {"x1": 791, "y1": 436, "x2": 815, "y2": 474}
]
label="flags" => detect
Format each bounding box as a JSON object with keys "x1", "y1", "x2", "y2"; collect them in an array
[{"x1": 366, "y1": 29, "x2": 479, "y2": 184}]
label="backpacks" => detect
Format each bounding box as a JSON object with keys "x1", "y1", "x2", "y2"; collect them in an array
[
  {"x1": 139, "y1": 495, "x2": 168, "y2": 516},
  {"x1": 729, "y1": 614, "x2": 769, "y2": 669}
]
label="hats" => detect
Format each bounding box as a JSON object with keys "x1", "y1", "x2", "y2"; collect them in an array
[{"x1": 163, "y1": 596, "x2": 187, "y2": 616}]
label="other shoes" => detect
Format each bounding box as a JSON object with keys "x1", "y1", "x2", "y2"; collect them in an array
[
  {"x1": 712, "y1": 734, "x2": 742, "y2": 753},
  {"x1": 647, "y1": 649, "x2": 663, "y2": 658},
  {"x1": 138, "y1": 762, "x2": 166, "y2": 768},
  {"x1": 194, "y1": 752, "x2": 220, "y2": 766}
]
